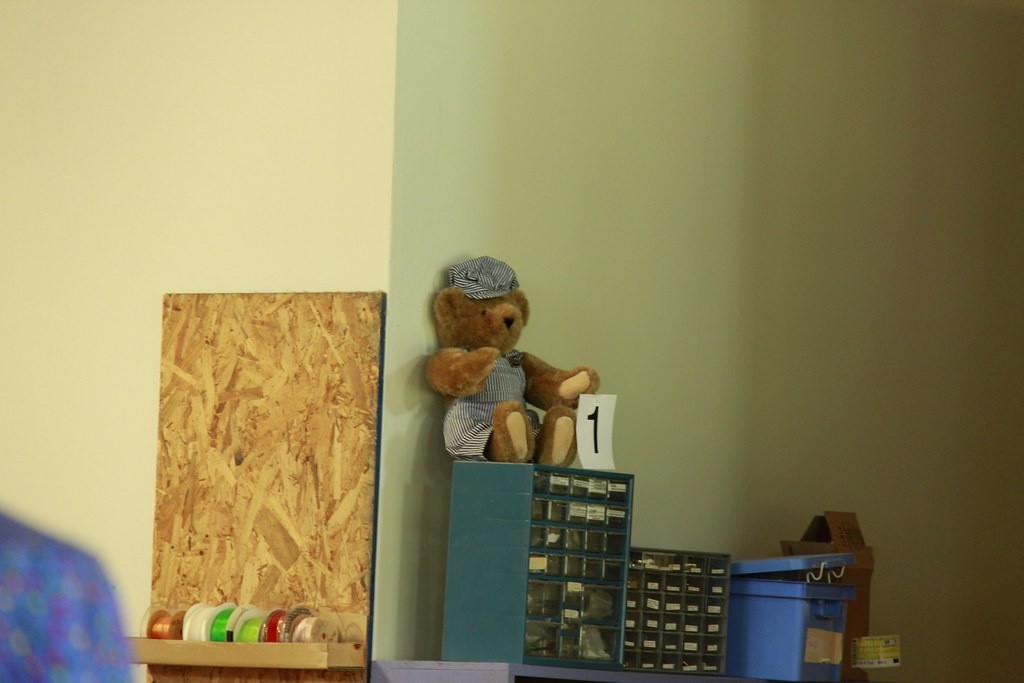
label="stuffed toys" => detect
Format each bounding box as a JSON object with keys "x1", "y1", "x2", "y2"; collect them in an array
[{"x1": 426, "y1": 256, "x2": 600, "y2": 466}]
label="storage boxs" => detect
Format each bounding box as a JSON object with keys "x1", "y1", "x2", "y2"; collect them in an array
[{"x1": 440, "y1": 459, "x2": 876, "y2": 683}]
